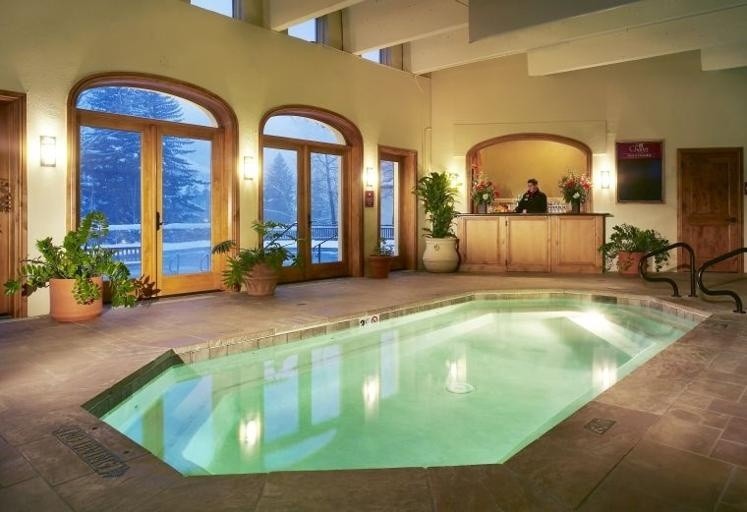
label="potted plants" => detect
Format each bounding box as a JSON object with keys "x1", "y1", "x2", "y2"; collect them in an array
[
  {"x1": 367, "y1": 235, "x2": 395, "y2": 278},
  {"x1": 210, "y1": 219, "x2": 311, "y2": 297},
  {"x1": 3, "y1": 211, "x2": 159, "y2": 321},
  {"x1": 597, "y1": 224, "x2": 670, "y2": 277},
  {"x1": 410, "y1": 168, "x2": 466, "y2": 272}
]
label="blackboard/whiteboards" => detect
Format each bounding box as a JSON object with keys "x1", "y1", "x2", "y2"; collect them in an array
[{"x1": 615, "y1": 138, "x2": 666, "y2": 204}]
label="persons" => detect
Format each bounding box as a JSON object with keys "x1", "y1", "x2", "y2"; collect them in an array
[{"x1": 507, "y1": 177, "x2": 548, "y2": 214}]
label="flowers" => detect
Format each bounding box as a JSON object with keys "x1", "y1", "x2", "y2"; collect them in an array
[
  {"x1": 467, "y1": 181, "x2": 499, "y2": 204},
  {"x1": 558, "y1": 168, "x2": 593, "y2": 204}
]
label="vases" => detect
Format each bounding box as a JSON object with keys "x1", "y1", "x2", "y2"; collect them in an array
[
  {"x1": 477, "y1": 201, "x2": 488, "y2": 214},
  {"x1": 570, "y1": 199, "x2": 581, "y2": 213}
]
